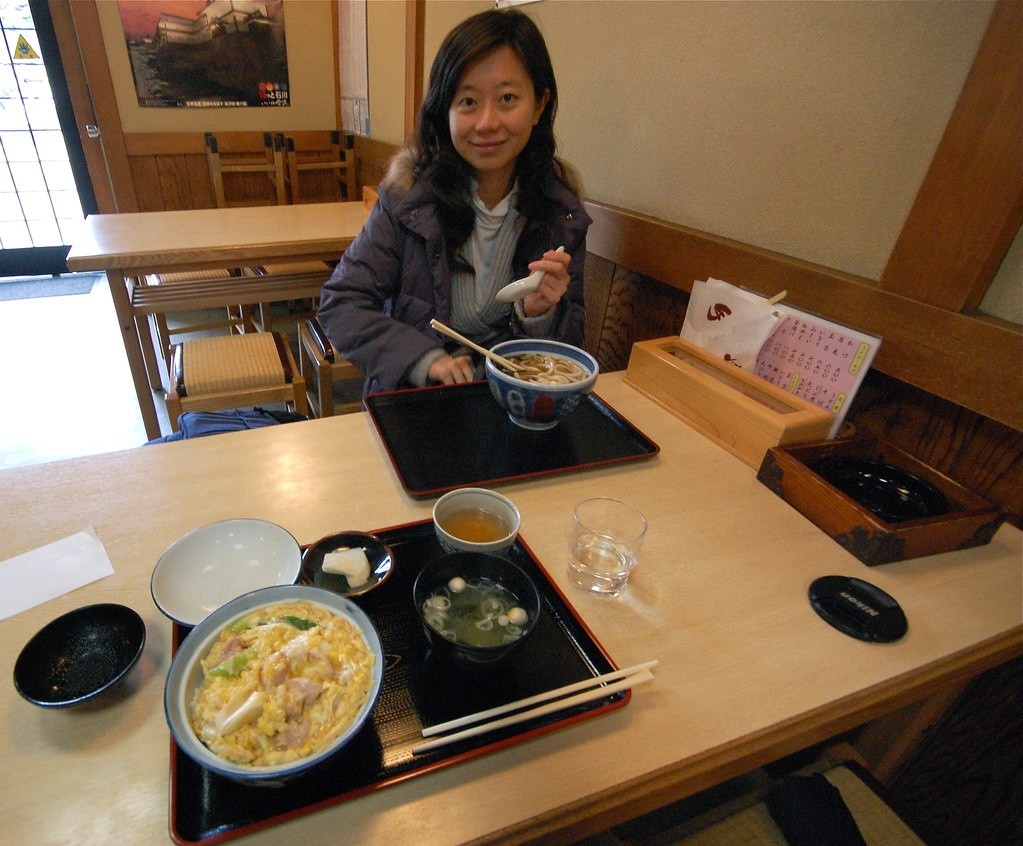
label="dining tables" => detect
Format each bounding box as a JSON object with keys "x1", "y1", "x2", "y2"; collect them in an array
[{"x1": 0, "y1": 368, "x2": 1023, "y2": 846}]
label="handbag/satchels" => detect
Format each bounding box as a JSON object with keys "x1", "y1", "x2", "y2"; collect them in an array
[{"x1": 140, "y1": 406, "x2": 308, "y2": 446}]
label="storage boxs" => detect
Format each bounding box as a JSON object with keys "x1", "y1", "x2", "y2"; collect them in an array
[
  {"x1": 620, "y1": 334, "x2": 836, "y2": 471},
  {"x1": 363, "y1": 185, "x2": 378, "y2": 210},
  {"x1": 757, "y1": 433, "x2": 1013, "y2": 568}
]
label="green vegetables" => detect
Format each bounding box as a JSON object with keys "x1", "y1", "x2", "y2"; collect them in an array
[{"x1": 204, "y1": 615, "x2": 316, "y2": 677}]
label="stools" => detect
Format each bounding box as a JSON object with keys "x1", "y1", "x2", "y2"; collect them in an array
[{"x1": 146, "y1": 130, "x2": 368, "y2": 432}]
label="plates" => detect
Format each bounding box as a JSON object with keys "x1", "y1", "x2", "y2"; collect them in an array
[
  {"x1": 151, "y1": 518, "x2": 301, "y2": 628},
  {"x1": 301, "y1": 531, "x2": 394, "y2": 597},
  {"x1": 13, "y1": 603, "x2": 146, "y2": 706}
]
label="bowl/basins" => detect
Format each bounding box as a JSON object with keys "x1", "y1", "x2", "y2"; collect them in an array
[
  {"x1": 412, "y1": 552, "x2": 540, "y2": 664},
  {"x1": 486, "y1": 339, "x2": 599, "y2": 430},
  {"x1": 432, "y1": 487, "x2": 522, "y2": 557},
  {"x1": 164, "y1": 584, "x2": 382, "y2": 789}
]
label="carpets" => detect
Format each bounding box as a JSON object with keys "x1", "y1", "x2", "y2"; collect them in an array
[{"x1": 0, "y1": 274, "x2": 98, "y2": 301}]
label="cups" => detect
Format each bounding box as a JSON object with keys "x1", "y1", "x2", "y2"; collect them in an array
[{"x1": 567, "y1": 498, "x2": 648, "y2": 597}]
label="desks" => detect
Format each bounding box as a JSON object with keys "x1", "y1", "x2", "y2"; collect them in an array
[{"x1": 67, "y1": 203, "x2": 371, "y2": 441}]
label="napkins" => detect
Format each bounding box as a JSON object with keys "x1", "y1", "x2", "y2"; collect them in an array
[{"x1": 0, "y1": 523, "x2": 115, "y2": 621}]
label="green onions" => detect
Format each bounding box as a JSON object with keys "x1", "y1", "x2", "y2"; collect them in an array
[{"x1": 425, "y1": 577, "x2": 523, "y2": 646}]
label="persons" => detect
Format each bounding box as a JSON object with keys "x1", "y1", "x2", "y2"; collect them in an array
[{"x1": 317, "y1": 7, "x2": 593, "y2": 411}]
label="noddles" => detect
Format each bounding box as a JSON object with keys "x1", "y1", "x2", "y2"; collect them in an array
[{"x1": 496, "y1": 353, "x2": 589, "y2": 386}]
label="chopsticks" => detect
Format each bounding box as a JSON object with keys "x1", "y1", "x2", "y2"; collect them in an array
[
  {"x1": 411, "y1": 659, "x2": 660, "y2": 755},
  {"x1": 430, "y1": 319, "x2": 526, "y2": 372}
]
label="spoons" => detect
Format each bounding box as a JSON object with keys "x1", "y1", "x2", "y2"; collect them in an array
[{"x1": 495, "y1": 246, "x2": 564, "y2": 303}]
